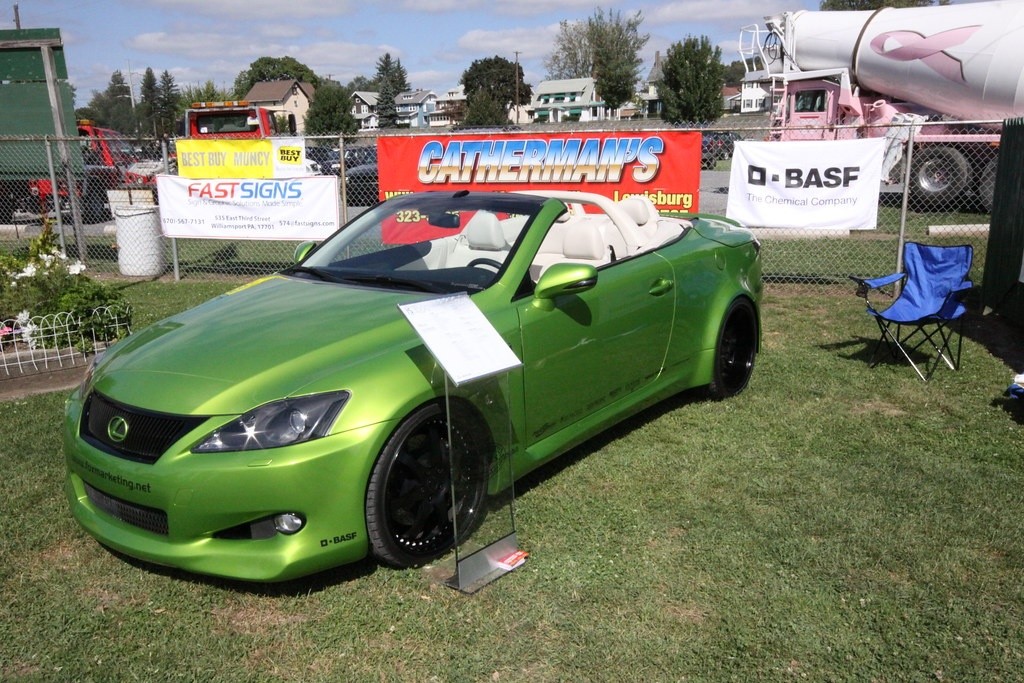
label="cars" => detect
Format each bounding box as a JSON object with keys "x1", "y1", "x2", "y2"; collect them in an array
[
  {"x1": 62, "y1": 186, "x2": 767, "y2": 585},
  {"x1": 701, "y1": 130, "x2": 732, "y2": 172},
  {"x1": 19, "y1": 84, "x2": 378, "y2": 210}
]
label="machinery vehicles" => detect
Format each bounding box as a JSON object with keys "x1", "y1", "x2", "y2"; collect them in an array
[{"x1": 732, "y1": 1, "x2": 1022, "y2": 216}]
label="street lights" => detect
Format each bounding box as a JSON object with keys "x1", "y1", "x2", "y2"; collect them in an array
[{"x1": 513, "y1": 48, "x2": 524, "y2": 124}]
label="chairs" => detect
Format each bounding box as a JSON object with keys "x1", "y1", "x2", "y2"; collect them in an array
[
  {"x1": 850, "y1": 242, "x2": 978, "y2": 385},
  {"x1": 443, "y1": 209, "x2": 512, "y2": 275},
  {"x1": 622, "y1": 193, "x2": 661, "y2": 248},
  {"x1": 529, "y1": 221, "x2": 608, "y2": 286}
]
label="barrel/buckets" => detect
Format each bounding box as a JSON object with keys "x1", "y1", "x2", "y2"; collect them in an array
[{"x1": 114, "y1": 206, "x2": 168, "y2": 275}]
